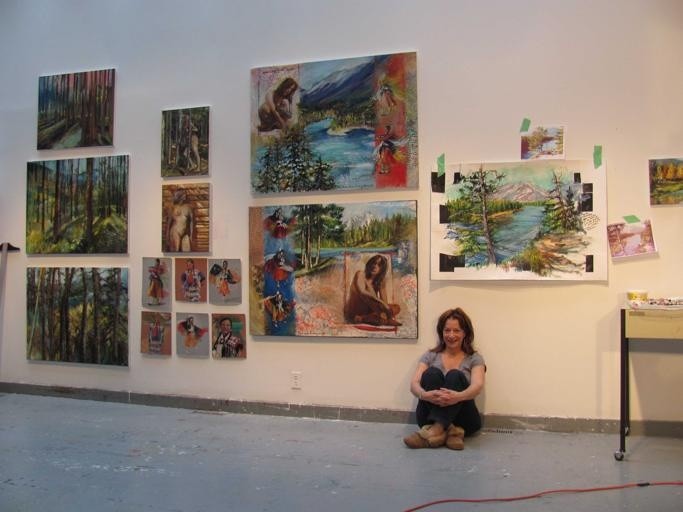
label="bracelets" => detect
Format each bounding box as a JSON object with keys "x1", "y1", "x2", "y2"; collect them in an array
[{"x1": 418, "y1": 389, "x2": 424, "y2": 398}]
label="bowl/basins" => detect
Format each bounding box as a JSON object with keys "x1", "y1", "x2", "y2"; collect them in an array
[{"x1": 626, "y1": 290, "x2": 648, "y2": 309}]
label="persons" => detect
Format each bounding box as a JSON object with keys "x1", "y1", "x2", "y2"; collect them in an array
[
  {"x1": 182, "y1": 113, "x2": 201, "y2": 171},
  {"x1": 374, "y1": 73, "x2": 397, "y2": 116},
  {"x1": 209, "y1": 260, "x2": 238, "y2": 298},
  {"x1": 262, "y1": 208, "x2": 296, "y2": 239},
  {"x1": 264, "y1": 247, "x2": 296, "y2": 289},
  {"x1": 346, "y1": 254, "x2": 402, "y2": 326},
  {"x1": 149, "y1": 317, "x2": 166, "y2": 354},
  {"x1": 178, "y1": 316, "x2": 203, "y2": 353},
  {"x1": 181, "y1": 259, "x2": 206, "y2": 302},
  {"x1": 264, "y1": 290, "x2": 296, "y2": 327},
  {"x1": 212, "y1": 318, "x2": 241, "y2": 358},
  {"x1": 166, "y1": 190, "x2": 194, "y2": 253},
  {"x1": 373, "y1": 125, "x2": 410, "y2": 174},
  {"x1": 146, "y1": 258, "x2": 164, "y2": 306},
  {"x1": 403, "y1": 307, "x2": 487, "y2": 451},
  {"x1": 256, "y1": 78, "x2": 298, "y2": 134}
]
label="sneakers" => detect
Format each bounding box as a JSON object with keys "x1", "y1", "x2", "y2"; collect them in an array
[
  {"x1": 446, "y1": 425, "x2": 464, "y2": 450},
  {"x1": 403, "y1": 424, "x2": 446, "y2": 448}
]
